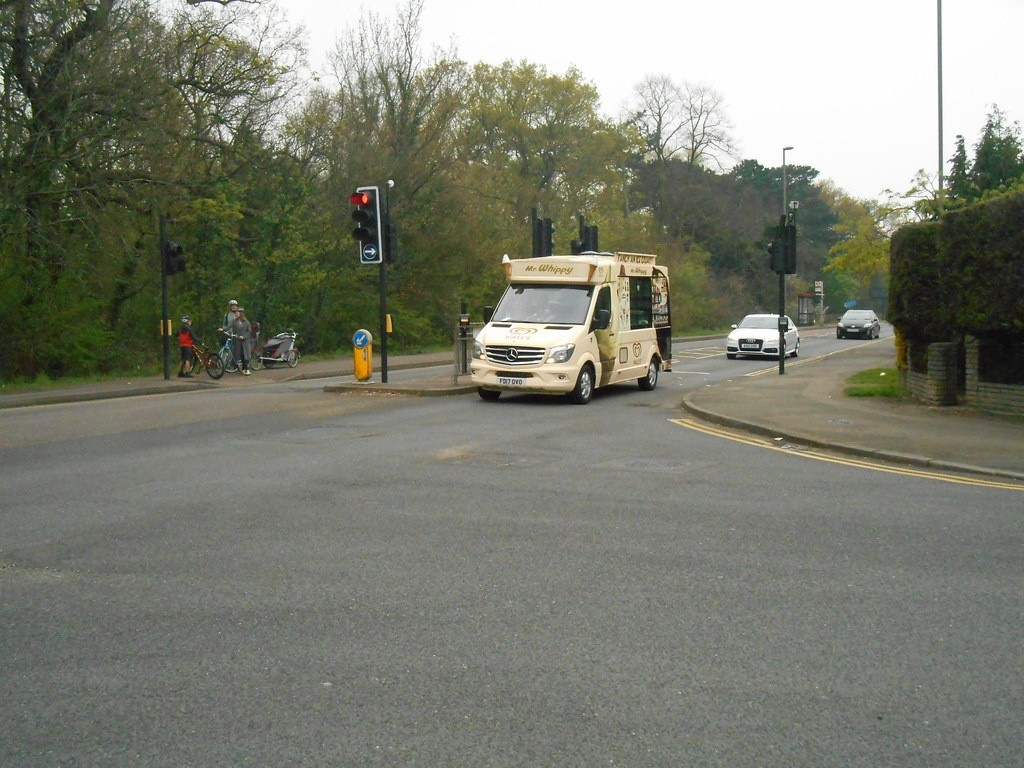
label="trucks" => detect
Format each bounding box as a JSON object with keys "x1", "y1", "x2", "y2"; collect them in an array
[{"x1": 469, "y1": 251, "x2": 672, "y2": 405}]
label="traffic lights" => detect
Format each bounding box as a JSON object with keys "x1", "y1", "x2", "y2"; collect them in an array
[
  {"x1": 166, "y1": 241, "x2": 186, "y2": 275},
  {"x1": 767, "y1": 242, "x2": 775, "y2": 270},
  {"x1": 350, "y1": 186, "x2": 382, "y2": 263},
  {"x1": 541, "y1": 218, "x2": 555, "y2": 257},
  {"x1": 570, "y1": 239, "x2": 583, "y2": 255}
]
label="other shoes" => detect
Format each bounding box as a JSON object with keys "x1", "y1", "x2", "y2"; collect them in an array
[
  {"x1": 177, "y1": 372, "x2": 183, "y2": 377},
  {"x1": 184, "y1": 372, "x2": 193, "y2": 378},
  {"x1": 242, "y1": 369, "x2": 251, "y2": 375}
]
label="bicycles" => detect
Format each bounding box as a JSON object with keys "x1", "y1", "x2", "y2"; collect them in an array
[
  {"x1": 216, "y1": 331, "x2": 252, "y2": 372},
  {"x1": 181, "y1": 339, "x2": 225, "y2": 380}
]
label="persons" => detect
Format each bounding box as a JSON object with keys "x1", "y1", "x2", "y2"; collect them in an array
[
  {"x1": 174, "y1": 316, "x2": 204, "y2": 378},
  {"x1": 223, "y1": 300, "x2": 239, "y2": 328},
  {"x1": 218, "y1": 307, "x2": 251, "y2": 376}
]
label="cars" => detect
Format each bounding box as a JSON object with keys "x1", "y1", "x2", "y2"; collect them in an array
[
  {"x1": 726, "y1": 314, "x2": 800, "y2": 361},
  {"x1": 836, "y1": 310, "x2": 880, "y2": 340}
]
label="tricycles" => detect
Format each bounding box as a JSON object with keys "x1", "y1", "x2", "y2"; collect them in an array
[{"x1": 225, "y1": 321, "x2": 301, "y2": 372}]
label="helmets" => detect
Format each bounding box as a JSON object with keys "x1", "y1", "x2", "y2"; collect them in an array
[
  {"x1": 229, "y1": 300, "x2": 238, "y2": 307},
  {"x1": 181, "y1": 316, "x2": 189, "y2": 323},
  {"x1": 235, "y1": 307, "x2": 245, "y2": 312}
]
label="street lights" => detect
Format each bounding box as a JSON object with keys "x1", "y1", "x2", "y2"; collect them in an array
[{"x1": 783, "y1": 147, "x2": 793, "y2": 226}]
